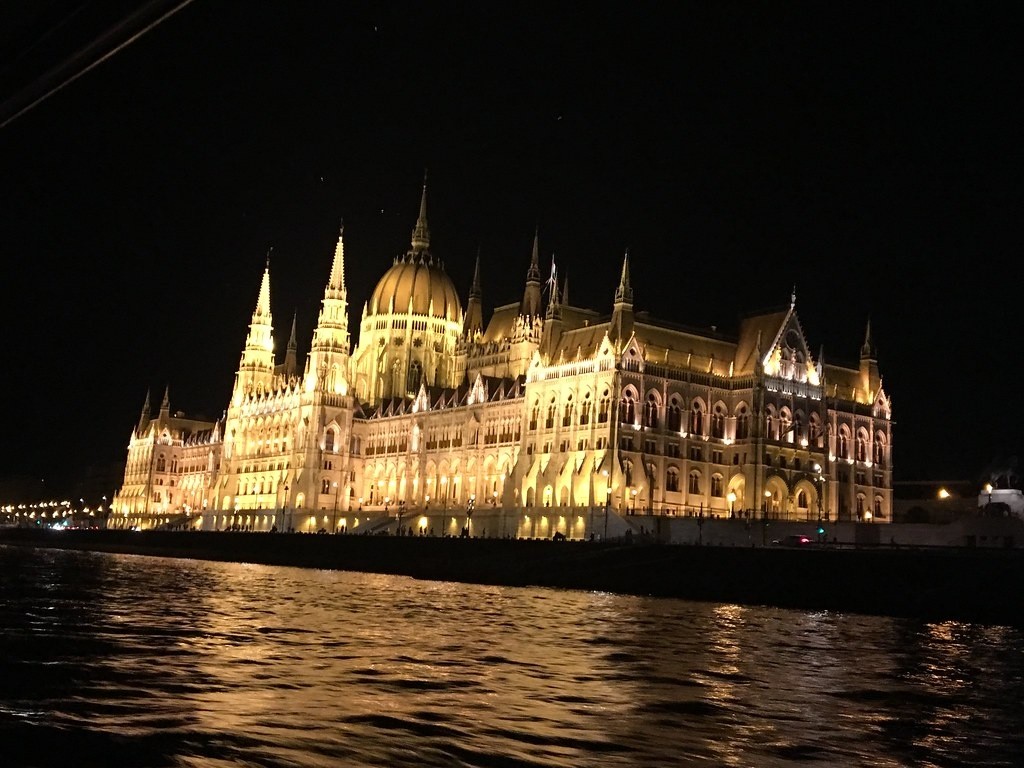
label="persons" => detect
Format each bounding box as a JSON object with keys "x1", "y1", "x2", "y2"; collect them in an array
[{"x1": 105, "y1": 520, "x2": 658, "y2": 541}]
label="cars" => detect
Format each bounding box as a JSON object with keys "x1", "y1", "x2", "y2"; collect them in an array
[{"x1": 772, "y1": 534, "x2": 818, "y2": 548}]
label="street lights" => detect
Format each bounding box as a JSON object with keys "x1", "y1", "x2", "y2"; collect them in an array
[
  {"x1": 727, "y1": 488, "x2": 737, "y2": 519},
  {"x1": 467, "y1": 498, "x2": 475, "y2": 538},
  {"x1": 397, "y1": 500, "x2": 405, "y2": 536},
  {"x1": 812, "y1": 464, "x2": 826, "y2": 520},
  {"x1": 333, "y1": 481, "x2": 339, "y2": 534},
  {"x1": 984, "y1": 482, "x2": 993, "y2": 503},
  {"x1": 252, "y1": 486, "x2": 262, "y2": 533},
  {"x1": 632, "y1": 489, "x2": 637, "y2": 510},
  {"x1": 440, "y1": 476, "x2": 448, "y2": 538},
  {"x1": 1, "y1": 496, "x2": 107, "y2": 530},
  {"x1": 602, "y1": 468, "x2": 612, "y2": 540},
  {"x1": 281, "y1": 486, "x2": 289, "y2": 533}
]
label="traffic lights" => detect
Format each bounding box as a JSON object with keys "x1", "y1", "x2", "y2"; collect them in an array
[{"x1": 816, "y1": 519, "x2": 825, "y2": 534}]
